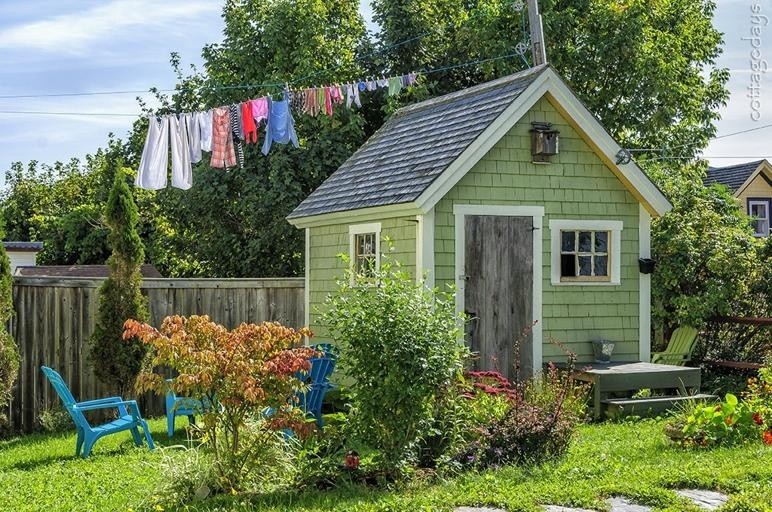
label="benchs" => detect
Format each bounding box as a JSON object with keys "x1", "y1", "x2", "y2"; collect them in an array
[{"x1": 602, "y1": 393, "x2": 719, "y2": 421}]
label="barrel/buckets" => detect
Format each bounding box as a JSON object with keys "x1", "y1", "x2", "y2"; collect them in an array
[{"x1": 590, "y1": 339, "x2": 615, "y2": 363}]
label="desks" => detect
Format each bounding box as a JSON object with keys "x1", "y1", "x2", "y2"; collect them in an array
[{"x1": 542, "y1": 360, "x2": 702, "y2": 422}]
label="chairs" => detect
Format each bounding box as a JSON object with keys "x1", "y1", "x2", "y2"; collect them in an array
[
  {"x1": 40, "y1": 364, "x2": 155, "y2": 459},
  {"x1": 651, "y1": 324, "x2": 700, "y2": 366},
  {"x1": 259, "y1": 342, "x2": 340, "y2": 451},
  {"x1": 164, "y1": 378, "x2": 223, "y2": 436}
]
